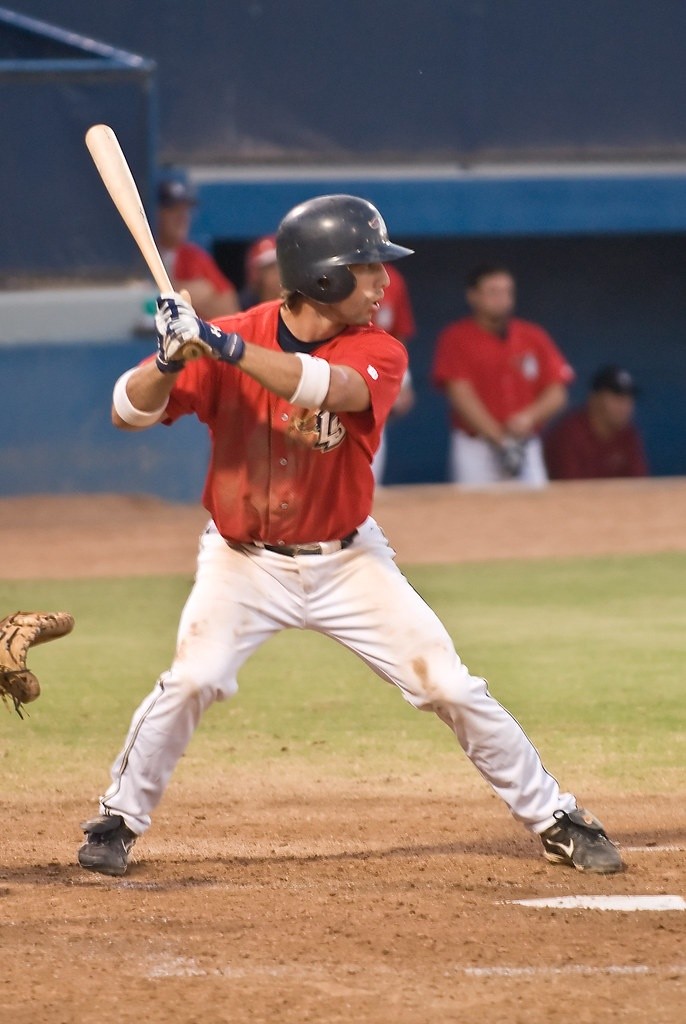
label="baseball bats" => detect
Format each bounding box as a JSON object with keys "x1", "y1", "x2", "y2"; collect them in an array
[{"x1": 85, "y1": 124, "x2": 203, "y2": 360}]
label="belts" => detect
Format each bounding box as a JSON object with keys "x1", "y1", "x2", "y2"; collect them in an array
[{"x1": 249, "y1": 529, "x2": 358, "y2": 556}]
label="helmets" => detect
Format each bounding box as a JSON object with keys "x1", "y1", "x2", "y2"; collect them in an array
[{"x1": 274, "y1": 195, "x2": 416, "y2": 306}]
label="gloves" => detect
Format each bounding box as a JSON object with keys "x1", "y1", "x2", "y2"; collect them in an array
[
  {"x1": 155, "y1": 290, "x2": 196, "y2": 370},
  {"x1": 164, "y1": 313, "x2": 246, "y2": 364}
]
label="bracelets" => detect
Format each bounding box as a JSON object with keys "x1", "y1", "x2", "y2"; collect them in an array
[
  {"x1": 112, "y1": 366, "x2": 170, "y2": 427},
  {"x1": 287, "y1": 351, "x2": 331, "y2": 412}
]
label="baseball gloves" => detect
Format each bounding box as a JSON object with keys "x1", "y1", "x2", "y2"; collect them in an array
[{"x1": 0, "y1": 610, "x2": 75, "y2": 720}]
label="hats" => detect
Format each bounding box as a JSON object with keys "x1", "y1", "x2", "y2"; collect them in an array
[
  {"x1": 159, "y1": 184, "x2": 200, "y2": 208},
  {"x1": 591, "y1": 367, "x2": 638, "y2": 393}
]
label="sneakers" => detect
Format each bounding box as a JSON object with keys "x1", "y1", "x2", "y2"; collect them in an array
[
  {"x1": 540, "y1": 808, "x2": 629, "y2": 875},
  {"x1": 76, "y1": 815, "x2": 137, "y2": 876}
]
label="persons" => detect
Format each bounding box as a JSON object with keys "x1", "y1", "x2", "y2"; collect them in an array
[
  {"x1": 433, "y1": 260, "x2": 574, "y2": 492},
  {"x1": 249, "y1": 232, "x2": 413, "y2": 486},
  {"x1": 158, "y1": 180, "x2": 238, "y2": 324},
  {"x1": 0, "y1": 610, "x2": 76, "y2": 705},
  {"x1": 542, "y1": 367, "x2": 647, "y2": 481},
  {"x1": 77, "y1": 192, "x2": 627, "y2": 872}
]
